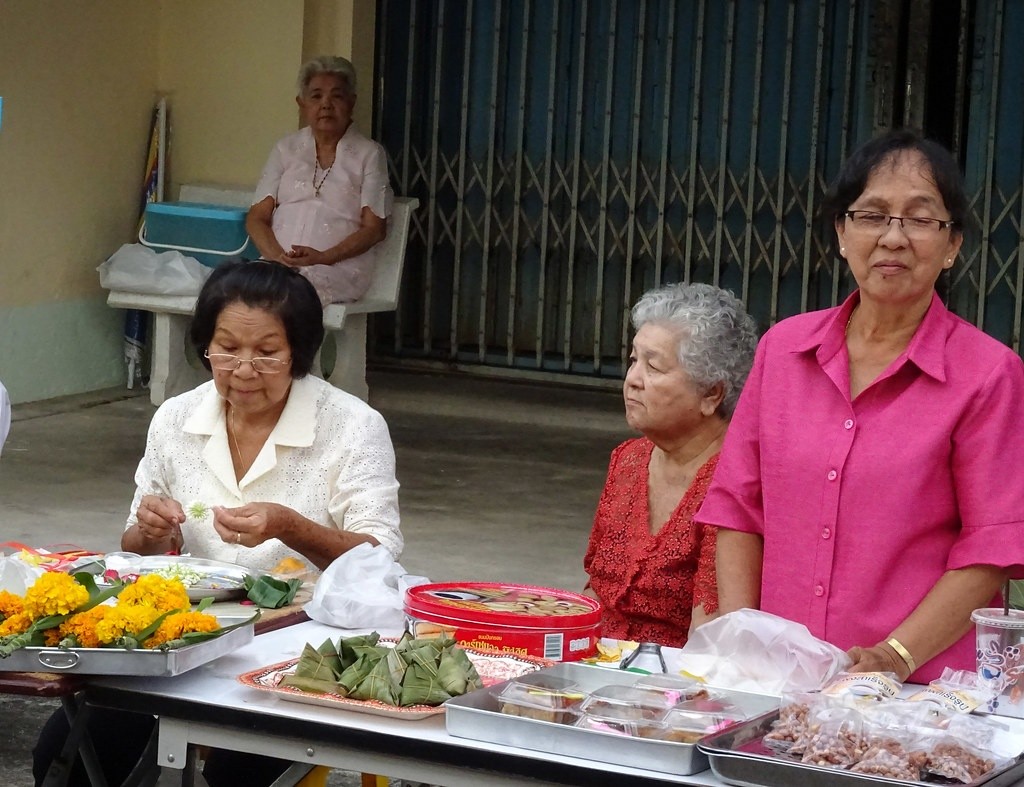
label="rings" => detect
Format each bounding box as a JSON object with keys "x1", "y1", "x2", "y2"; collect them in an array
[{"x1": 238, "y1": 534, "x2": 240, "y2": 542}]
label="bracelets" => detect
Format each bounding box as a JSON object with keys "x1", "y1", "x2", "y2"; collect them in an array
[{"x1": 885, "y1": 637, "x2": 916, "y2": 675}]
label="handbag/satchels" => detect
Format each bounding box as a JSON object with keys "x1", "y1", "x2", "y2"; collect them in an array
[{"x1": 95, "y1": 243, "x2": 215, "y2": 296}]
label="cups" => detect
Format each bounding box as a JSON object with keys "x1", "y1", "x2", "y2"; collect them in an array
[
  {"x1": 970, "y1": 608, "x2": 1024, "y2": 713},
  {"x1": 103, "y1": 551, "x2": 143, "y2": 578}
]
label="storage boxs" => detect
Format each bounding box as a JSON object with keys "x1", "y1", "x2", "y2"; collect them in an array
[
  {"x1": 402, "y1": 582, "x2": 603, "y2": 661},
  {"x1": 139, "y1": 202, "x2": 259, "y2": 270}
]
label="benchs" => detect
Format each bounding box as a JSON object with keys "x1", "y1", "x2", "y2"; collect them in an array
[{"x1": 106, "y1": 187, "x2": 420, "y2": 407}]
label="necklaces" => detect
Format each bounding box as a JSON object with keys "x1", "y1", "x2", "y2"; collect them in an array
[
  {"x1": 313, "y1": 156, "x2": 335, "y2": 196},
  {"x1": 844, "y1": 305, "x2": 859, "y2": 335},
  {"x1": 232, "y1": 411, "x2": 246, "y2": 472}
]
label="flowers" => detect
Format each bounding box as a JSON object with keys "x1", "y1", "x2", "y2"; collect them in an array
[{"x1": 0, "y1": 567, "x2": 222, "y2": 659}]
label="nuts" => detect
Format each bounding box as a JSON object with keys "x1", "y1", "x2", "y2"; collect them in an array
[{"x1": 763, "y1": 702, "x2": 996, "y2": 783}]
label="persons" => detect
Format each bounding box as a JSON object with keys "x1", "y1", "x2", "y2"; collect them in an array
[
  {"x1": 247, "y1": 56, "x2": 396, "y2": 302},
  {"x1": 695, "y1": 126, "x2": 1024, "y2": 680},
  {"x1": 584, "y1": 283, "x2": 759, "y2": 647},
  {"x1": 32, "y1": 259, "x2": 402, "y2": 787}
]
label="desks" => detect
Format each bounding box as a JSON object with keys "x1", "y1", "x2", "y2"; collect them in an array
[
  {"x1": 90, "y1": 612, "x2": 820, "y2": 787},
  {"x1": 0, "y1": 573, "x2": 312, "y2": 787}
]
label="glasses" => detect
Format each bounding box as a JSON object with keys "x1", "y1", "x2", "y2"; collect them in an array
[
  {"x1": 204, "y1": 348, "x2": 292, "y2": 374},
  {"x1": 845, "y1": 209, "x2": 954, "y2": 241}
]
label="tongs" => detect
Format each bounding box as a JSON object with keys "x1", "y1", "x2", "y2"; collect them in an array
[{"x1": 619, "y1": 642, "x2": 668, "y2": 674}]
